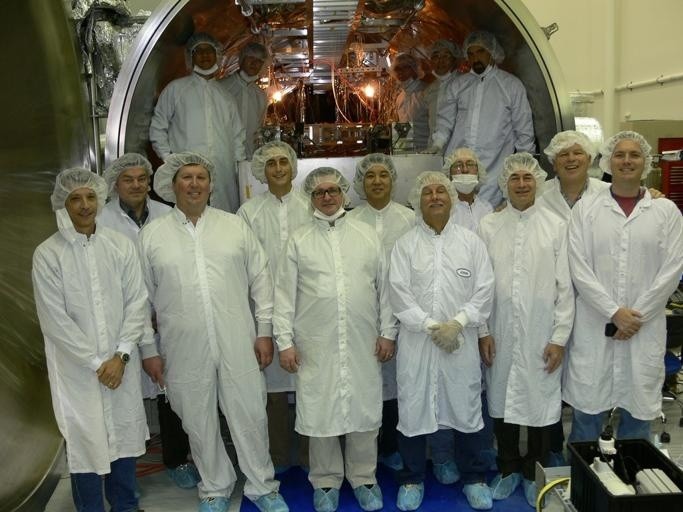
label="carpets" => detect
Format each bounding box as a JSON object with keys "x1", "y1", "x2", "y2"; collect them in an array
[{"x1": 239, "y1": 456, "x2": 552, "y2": 512}]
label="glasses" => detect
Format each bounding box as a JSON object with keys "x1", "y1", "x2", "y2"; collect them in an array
[
  {"x1": 191, "y1": 47, "x2": 214, "y2": 55},
  {"x1": 310, "y1": 187, "x2": 342, "y2": 199},
  {"x1": 450, "y1": 160, "x2": 477, "y2": 170}
]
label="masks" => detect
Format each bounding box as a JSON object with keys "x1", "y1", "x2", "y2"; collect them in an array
[
  {"x1": 239, "y1": 69, "x2": 260, "y2": 83},
  {"x1": 53, "y1": 208, "x2": 79, "y2": 244},
  {"x1": 450, "y1": 174, "x2": 479, "y2": 195},
  {"x1": 310, "y1": 202, "x2": 345, "y2": 222},
  {"x1": 431, "y1": 68, "x2": 452, "y2": 79},
  {"x1": 469, "y1": 61, "x2": 492, "y2": 77},
  {"x1": 395, "y1": 77, "x2": 413, "y2": 87},
  {"x1": 192, "y1": 63, "x2": 218, "y2": 75}
]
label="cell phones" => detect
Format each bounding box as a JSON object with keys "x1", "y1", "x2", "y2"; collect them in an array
[{"x1": 604, "y1": 322, "x2": 617, "y2": 336}]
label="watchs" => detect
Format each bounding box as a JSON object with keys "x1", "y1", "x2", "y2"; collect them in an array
[{"x1": 114, "y1": 352, "x2": 129, "y2": 365}]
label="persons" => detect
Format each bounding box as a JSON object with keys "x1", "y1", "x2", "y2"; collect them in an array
[
  {"x1": 561, "y1": 131, "x2": 683, "y2": 464},
  {"x1": 136, "y1": 152, "x2": 289, "y2": 512},
  {"x1": 32, "y1": 167, "x2": 149, "y2": 512},
  {"x1": 272, "y1": 168, "x2": 399, "y2": 512},
  {"x1": 479, "y1": 152, "x2": 576, "y2": 509},
  {"x1": 347, "y1": 153, "x2": 416, "y2": 473},
  {"x1": 427, "y1": 147, "x2": 498, "y2": 485},
  {"x1": 423, "y1": 38, "x2": 470, "y2": 155},
  {"x1": 493, "y1": 130, "x2": 666, "y2": 466},
  {"x1": 149, "y1": 31, "x2": 246, "y2": 213},
  {"x1": 389, "y1": 170, "x2": 496, "y2": 511},
  {"x1": 236, "y1": 141, "x2": 312, "y2": 473},
  {"x1": 420, "y1": 30, "x2": 536, "y2": 208},
  {"x1": 96, "y1": 153, "x2": 201, "y2": 489},
  {"x1": 217, "y1": 41, "x2": 268, "y2": 161},
  {"x1": 392, "y1": 53, "x2": 429, "y2": 153}
]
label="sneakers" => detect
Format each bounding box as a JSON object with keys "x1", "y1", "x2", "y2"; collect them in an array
[
  {"x1": 460, "y1": 481, "x2": 494, "y2": 511},
  {"x1": 352, "y1": 482, "x2": 384, "y2": 512},
  {"x1": 164, "y1": 459, "x2": 201, "y2": 489},
  {"x1": 433, "y1": 458, "x2": 462, "y2": 485},
  {"x1": 521, "y1": 476, "x2": 538, "y2": 508},
  {"x1": 312, "y1": 485, "x2": 341, "y2": 512},
  {"x1": 251, "y1": 492, "x2": 290, "y2": 512},
  {"x1": 381, "y1": 451, "x2": 405, "y2": 472},
  {"x1": 488, "y1": 470, "x2": 522, "y2": 502},
  {"x1": 198, "y1": 494, "x2": 231, "y2": 512},
  {"x1": 395, "y1": 481, "x2": 426, "y2": 512}
]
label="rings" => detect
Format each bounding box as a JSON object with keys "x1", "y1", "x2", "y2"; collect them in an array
[{"x1": 109, "y1": 382, "x2": 113, "y2": 386}]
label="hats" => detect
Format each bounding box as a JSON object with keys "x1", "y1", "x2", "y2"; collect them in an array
[
  {"x1": 497, "y1": 152, "x2": 549, "y2": 200},
  {"x1": 408, "y1": 170, "x2": 458, "y2": 216},
  {"x1": 441, "y1": 147, "x2": 489, "y2": 193},
  {"x1": 393, "y1": 53, "x2": 417, "y2": 76},
  {"x1": 50, "y1": 168, "x2": 109, "y2": 218},
  {"x1": 462, "y1": 31, "x2": 506, "y2": 64},
  {"x1": 543, "y1": 129, "x2": 597, "y2": 165},
  {"x1": 184, "y1": 31, "x2": 224, "y2": 72},
  {"x1": 152, "y1": 151, "x2": 216, "y2": 204},
  {"x1": 302, "y1": 167, "x2": 351, "y2": 207},
  {"x1": 103, "y1": 152, "x2": 153, "y2": 198},
  {"x1": 429, "y1": 39, "x2": 460, "y2": 58},
  {"x1": 353, "y1": 152, "x2": 400, "y2": 200},
  {"x1": 240, "y1": 43, "x2": 268, "y2": 65},
  {"x1": 598, "y1": 131, "x2": 653, "y2": 181},
  {"x1": 250, "y1": 141, "x2": 299, "y2": 185}
]
